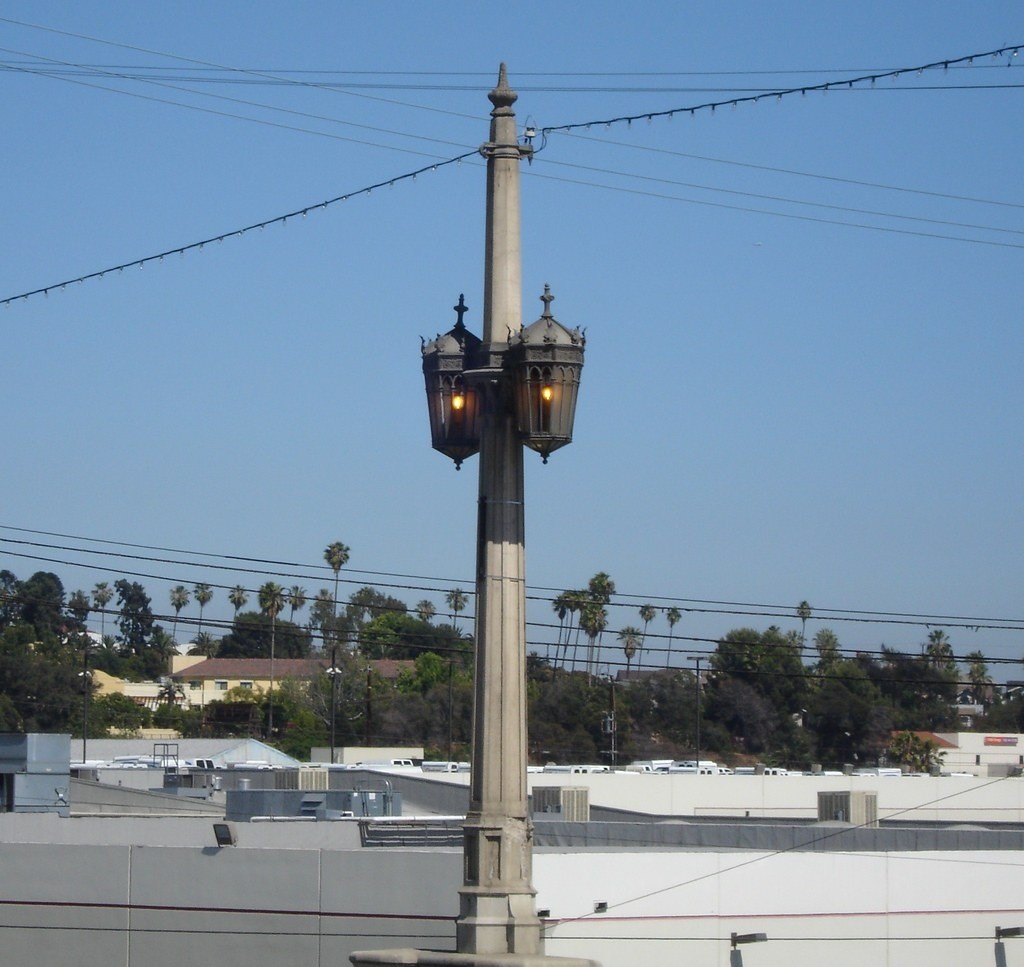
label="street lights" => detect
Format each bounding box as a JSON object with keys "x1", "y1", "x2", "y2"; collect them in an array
[
  {"x1": 324, "y1": 644, "x2": 343, "y2": 764},
  {"x1": 82, "y1": 649, "x2": 98, "y2": 765},
  {"x1": 417, "y1": 61, "x2": 590, "y2": 952},
  {"x1": 687, "y1": 656, "x2": 708, "y2": 768}
]
